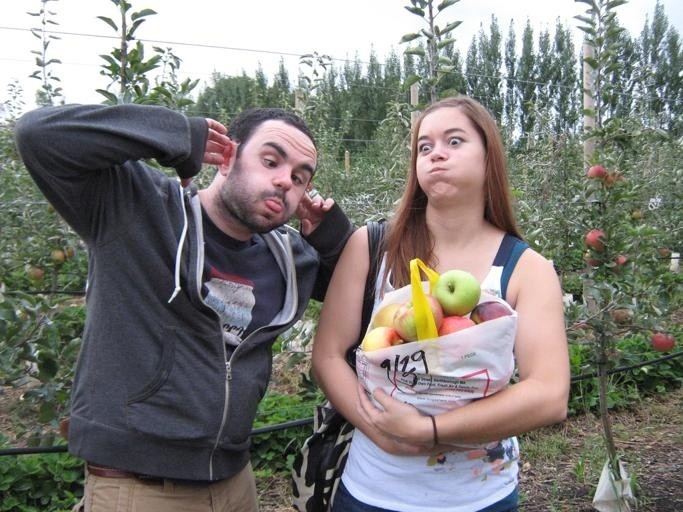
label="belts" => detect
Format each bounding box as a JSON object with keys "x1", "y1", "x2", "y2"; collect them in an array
[{"x1": 86, "y1": 465, "x2": 155, "y2": 480}]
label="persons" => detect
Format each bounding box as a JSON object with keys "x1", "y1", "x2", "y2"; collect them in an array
[
  {"x1": 13, "y1": 91, "x2": 360, "y2": 512},
  {"x1": 312, "y1": 96, "x2": 572, "y2": 512}
]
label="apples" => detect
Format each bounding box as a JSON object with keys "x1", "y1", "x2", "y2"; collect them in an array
[
  {"x1": 361, "y1": 271, "x2": 514, "y2": 354},
  {"x1": 583, "y1": 229, "x2": 628, "y2": 273},
  {"x1": 586, "y1": 162, "x2": 623, "y2": 189},
  {"x1": 651, "y1": 331, "x2": 674, "y2": 350},
  {"x1": 23, "y1": 247, "x2": 75, "y2": 281}
]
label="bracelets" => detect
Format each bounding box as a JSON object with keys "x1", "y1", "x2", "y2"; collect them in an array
[{"x1": 428, "y1": 415, "x2": 439, "y2": 446}]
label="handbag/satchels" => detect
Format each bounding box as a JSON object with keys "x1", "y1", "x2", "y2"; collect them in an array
[
  {"x1": 289, "y1": 216, "x2": 390, "y2": 511},
  {"x1": 355, "y1": 257, "x2": 519, "y2": 450}
]
